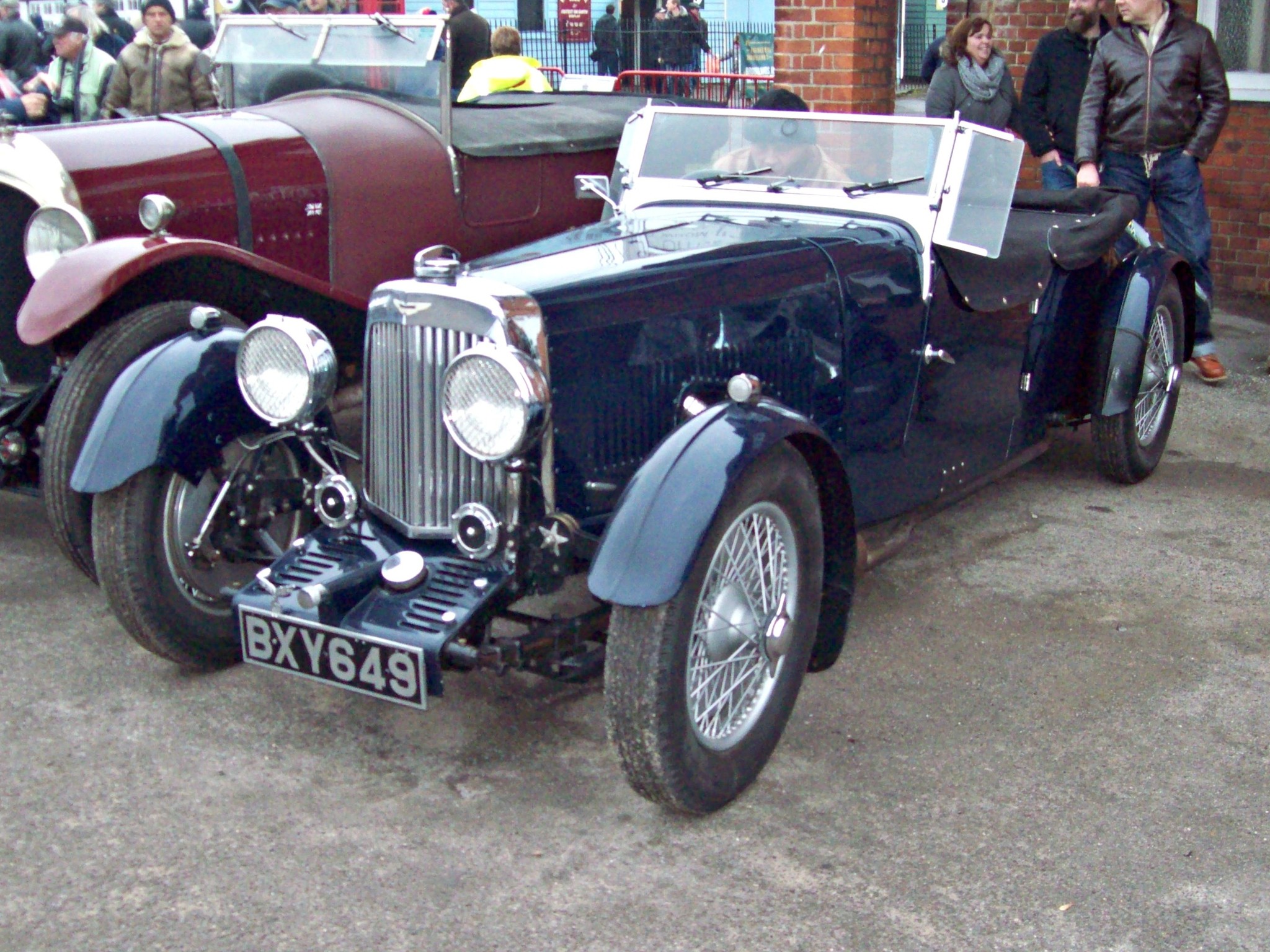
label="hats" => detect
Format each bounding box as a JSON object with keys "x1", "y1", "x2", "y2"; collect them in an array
[
  {"x1": 689, "y1": 2, "x2": 700, "y2": 8},
  {"x1": 44, "y1": 15, "x2": 88, "y2": 36},
  {"x1": 141, "y1": 0, "x2": 176, "y2": 24},
  {"x1": 655, "y1": 7, "x2": 666, "y2": 12}
]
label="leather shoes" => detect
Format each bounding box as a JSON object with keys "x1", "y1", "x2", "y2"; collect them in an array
[{"x1": 1192, "y1": 355, "x2": 1228, "y2": 382}]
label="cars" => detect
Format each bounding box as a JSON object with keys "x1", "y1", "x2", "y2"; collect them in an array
[
  {"x1": 69, "y1": 101, "x2": 1201, "y2": 818},
  {"x1": 0, "y1": 15, "x2": 815, "y2": 587}
]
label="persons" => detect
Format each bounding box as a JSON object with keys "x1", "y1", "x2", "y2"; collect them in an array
[
  {"x1": 1072, "y1": 0, "x2": 1233, "y2": 385},
  {"x1": 919, "y1": 28, "x2": 1021, "y2": 139},
  {"x1": 704, "y1": 87, "x2": 862, "y2": 190},
  {"x1": 592, "y1": 2, "x2": 623, "y2": 83},
  {"x1": 923, "y1": 14, "x2": 1018, "y2": 172},
  {"x1": 642, "y1": 0, "x2": 730, "y2": 104},
  {"x1": 455, "y1": 24, "x2": 555, "y2": 103},
  {"x1": 1014, "y1": 1, "x2": 1114, "y2": 197},
  {"x1": 0, "y1": 0, "x2": 494, "y2": 133}
]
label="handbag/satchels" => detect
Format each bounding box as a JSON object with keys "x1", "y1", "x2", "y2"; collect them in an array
[
  {"x1": 589, "y1": 49, "x2": 598, "y2": 62},
  {"x1": 706, "y1": 52, "x2": 720, "y2": 75}
]
